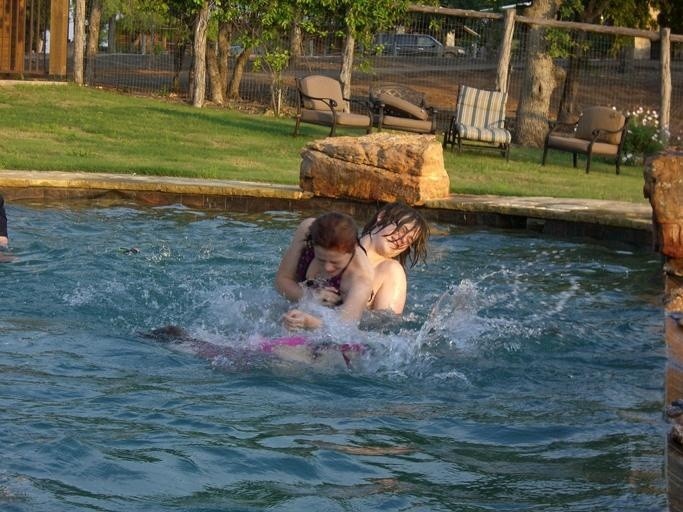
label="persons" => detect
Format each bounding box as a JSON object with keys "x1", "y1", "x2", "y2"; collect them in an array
[
  {"x1": 275, "y1": 211, "x2": 374, "y2": 371},
  {"x1": 355, "y1": 203, "x2": 432, "y2": 315},
  {"x1": 0, "y1": 194, "x2": 16, "y2": 263}
]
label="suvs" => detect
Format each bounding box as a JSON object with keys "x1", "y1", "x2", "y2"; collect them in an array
[{"x1": 371, "y1": 34, "x2": 466, "y2": 58}]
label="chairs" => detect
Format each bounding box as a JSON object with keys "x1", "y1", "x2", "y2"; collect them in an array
[
  {"x1": 441, "y1": 83, "x2": 512, "y2": 160},
  {"x1": 369, "y1": 83, "x2": 438, "y2": 135},
  {"x1": 542, "y1": 107, "x2": 632, "y2": 175},
  {"x1": 294, "y1": 74, "x2": 373, "y2": 137}
]
